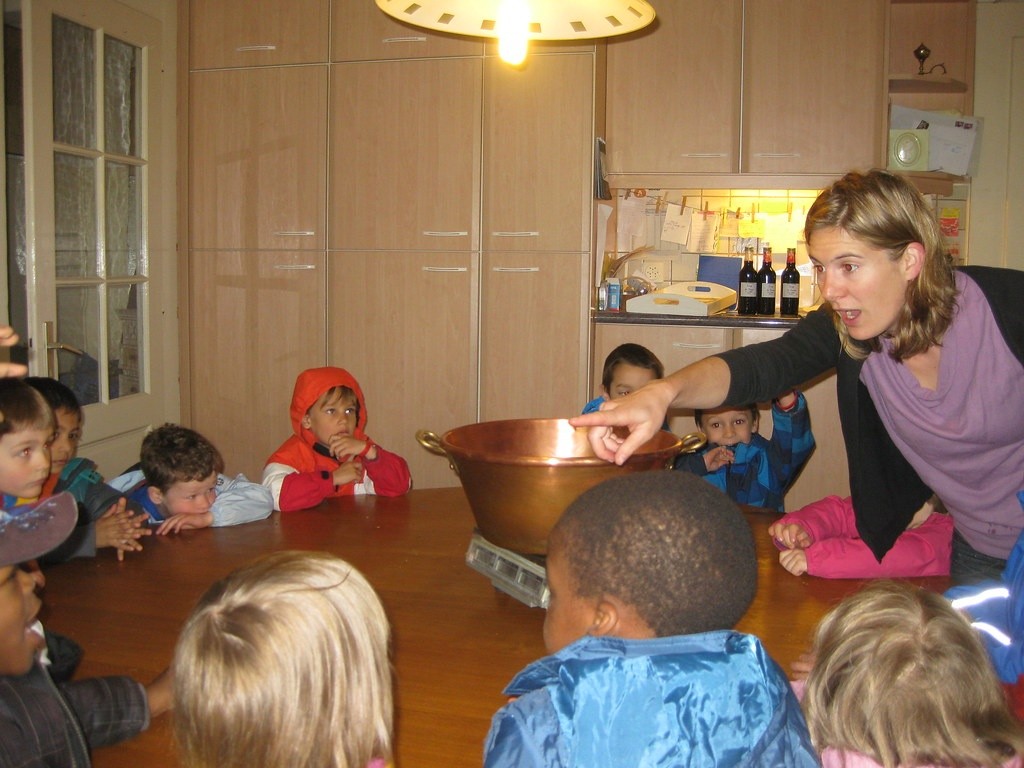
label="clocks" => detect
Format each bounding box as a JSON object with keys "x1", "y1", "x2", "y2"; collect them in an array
[{"x1": 885, "y1": 127, "x2": 930, "y2": 171}]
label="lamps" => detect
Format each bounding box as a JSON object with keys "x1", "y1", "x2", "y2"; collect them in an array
[{"x1": 374, "y1": 0, "x2": 656, "y2": 64}]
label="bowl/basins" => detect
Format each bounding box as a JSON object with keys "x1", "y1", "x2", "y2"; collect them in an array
[{"x1": 414, "y1": 421, "x2": 707, "y2": 557}]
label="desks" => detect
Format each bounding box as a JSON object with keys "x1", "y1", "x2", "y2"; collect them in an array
[{"x1": 33, "y1": 480, "x2": 959, "y2": 768}]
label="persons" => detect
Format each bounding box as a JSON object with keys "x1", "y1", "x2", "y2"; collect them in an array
[
  {"x1": 941, "y1": 490, "x2": 1024, "y2": 684},
  {"x1": 109, "y1": 425, "x2": 274, "y2": 536},
  {"x1": 582, "y1": 344, "x2": 669, "y2": 432},
  {"x1": 672, "y1": 384, "x2": 815, "y2": 509},
  {"x1": 0, "y1": 326, "x2": 152, "y2": 589},
  {"x1": 789, "y1": 582, "x2": 1024, "y2": 768},
  {"x1": 0, "y1": 492, "x2": 397, "y2": 768},
  {"x1": 263, "y1": 367, "x2": 413, "y2": 511},
  {"x1": 568, "y1": 169, "x2": 1024, "y2": 591},
  {"x1": 482, "y1": 471, "x2": 824, "y2": 768},
  {"x1": 768, "y1": 486, "x2": 954, "y2": 578}
]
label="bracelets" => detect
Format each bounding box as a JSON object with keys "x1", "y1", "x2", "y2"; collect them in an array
[{"x1": 359, "y1": 442, "x2": 371, "y2": 456}]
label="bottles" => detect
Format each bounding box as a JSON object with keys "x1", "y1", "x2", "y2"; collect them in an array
[
  {"x1": 738, "y1": 247, "x2": 757, "y2": 315},
  {"x1": 756, "y1": 246, "x2": 776, "y2": 315},
  {"x1": 780, "y1": 247, "x2": 801, "y2": 316}
]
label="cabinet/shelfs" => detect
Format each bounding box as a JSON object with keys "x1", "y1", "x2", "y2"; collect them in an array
[
  {"x1": 607, "y1": 0, "x2": 891, "y2": 190},
  {"x1": 178, "y1": 1, "x2": 595, "y2": 492},
  {"x1": 590, "y1": 320, "x2": 851, "y2": 513}
]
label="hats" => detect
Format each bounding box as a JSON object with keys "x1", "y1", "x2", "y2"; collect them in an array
[{"x1": 0, "y1": 493, "x2": 76, "y2": 565}]
